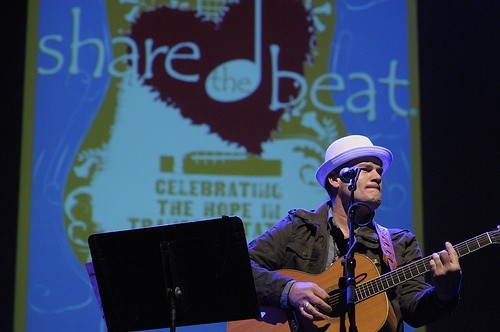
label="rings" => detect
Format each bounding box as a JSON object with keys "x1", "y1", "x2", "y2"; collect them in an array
[{"x1": 304, "y1": 302, "x2": 309, "y2": 309}]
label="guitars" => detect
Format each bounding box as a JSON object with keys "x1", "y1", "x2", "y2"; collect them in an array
[{"x1": 226, "y1": 226, "x2": 500, "y2": 332}]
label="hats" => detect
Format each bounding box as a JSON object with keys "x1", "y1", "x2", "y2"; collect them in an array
[{"x1": 317, "y1": 135, "x2": 394, "y2": 186}]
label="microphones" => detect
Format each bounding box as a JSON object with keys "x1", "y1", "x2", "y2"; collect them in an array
[{"x1": 340, "y1": 167, "x2": 360, "y2": 185}]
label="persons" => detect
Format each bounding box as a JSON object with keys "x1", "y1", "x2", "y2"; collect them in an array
[{"x1": 247, "y1": 135, "x2": 464, "y2": 332}]
